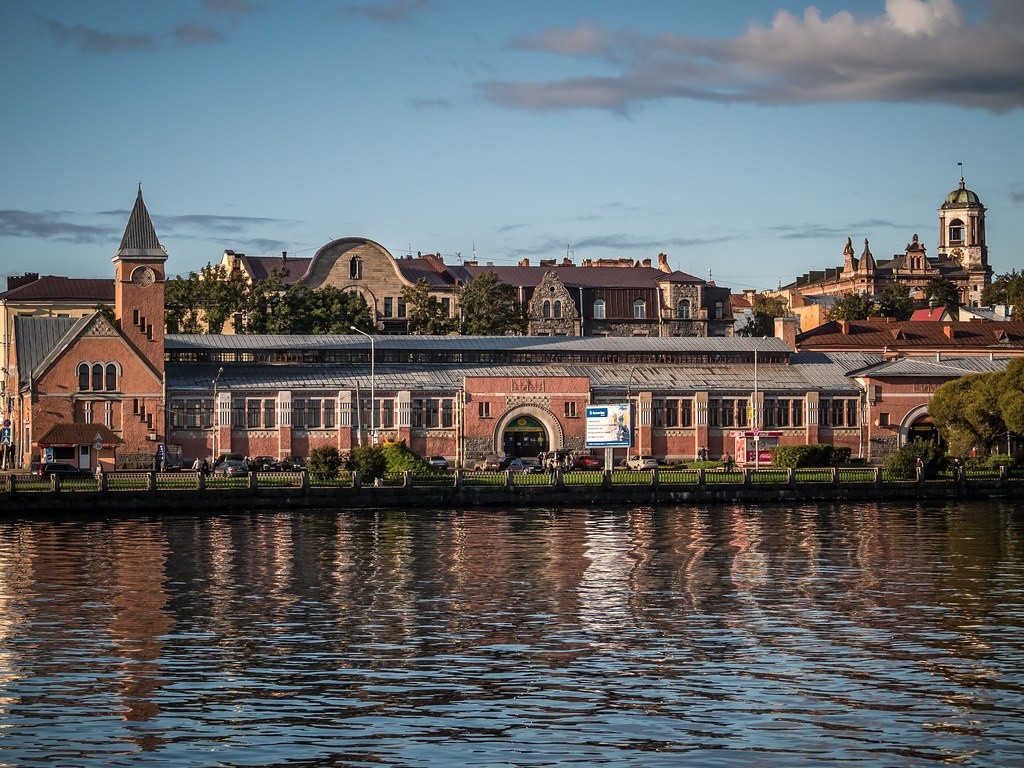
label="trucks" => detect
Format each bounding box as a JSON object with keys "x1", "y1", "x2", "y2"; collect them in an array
[{"x1": 153, "y1": 443, "x2": 183, "y2": 473}]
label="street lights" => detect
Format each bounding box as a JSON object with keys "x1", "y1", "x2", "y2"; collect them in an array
[
  {"x1": 454, "y1": 392, "x2": 459, "y2": 468},
  {"x1": 755, "y1": 335, "x2": 768, "y2": 472},
  {"x1": 212, "y1": 367, "x2": 223, "y2": 467},
  {"x1": 350, "y1": 326, "x2": 374, "y2": 447}
]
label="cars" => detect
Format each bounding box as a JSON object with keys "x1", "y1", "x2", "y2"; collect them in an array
[
  {"x1": 30, "y1": 463, "x2": 95, "y2": 481},
  {"x1": 424, "y1": 456, "x2": 448, "y2": 470},
  {"x1": 573, "y1": 455, "x2": 601, "y2": 470},
  {"x1": 215, "y1": 453, "x2": 244, "y2": 467},
  {"x1": 505, "y1": 458, "x2": 545, "y2": 475},
  {"x1": 626, "y1": 455, "x2": 658, "y2": 471},
  {"x1": 541, "y1": 451, "x2": 574, "y2": 471},
  {"x1": 214, "y1": 461, "x2": 249, "y2": 478},
  {"x1": 282, "y1": 456, "x2": 308, "y2": 471},
  {"x1": 474, "y1": 455, "x2": 500, "y2": 472},
  {"x1": 248, "y1": 456, "x2": 281, "y2": 472},
  {"x1": 489, "y1": 457, "x2": 515, "y2": 472}
]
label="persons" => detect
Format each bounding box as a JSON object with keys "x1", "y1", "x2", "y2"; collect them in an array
[
  {"x1": 915, "y1": 458, "x2": 923, "y2": 481},
  {"x1": 6, "y1": 442, "x2": 16, "y2": 463},
  {"x1": 537, "y1": 452, "x2": 571, "y2": 475},
  {"x1": 192, "y1": 457, "x2": 210, "y2": 477},
  {"x1": 698, "y1": 446, "x2": 709, "y2": 460},
  {"x1": 723, "y1": 452, "x2": 732, "y2": 471},
  {"x1": 242, "y1": 456, "x2": 255, "y2": 466},
  {"x1": 953, "y1": 459, "x2": 962, "y2": 481}
]
